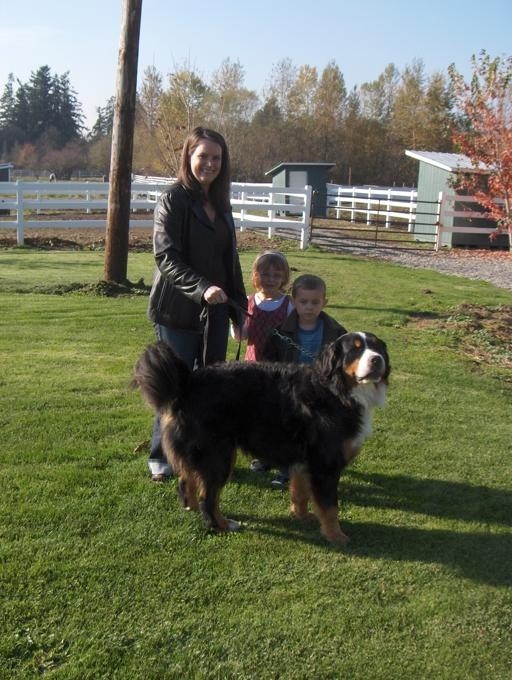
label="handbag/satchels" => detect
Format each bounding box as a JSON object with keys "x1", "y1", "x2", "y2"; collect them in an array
[{"x1": 147, "y1": 270, "x2": 207, "y2": 333}]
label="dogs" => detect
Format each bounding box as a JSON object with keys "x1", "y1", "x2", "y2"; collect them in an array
[{"x1": 128, "y1": 330, "x2": 392, "y2": 545}]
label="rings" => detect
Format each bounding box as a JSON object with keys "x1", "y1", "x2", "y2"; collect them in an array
[{"x1": 213, "y1": 301, "x2": 218, "y2": 305}]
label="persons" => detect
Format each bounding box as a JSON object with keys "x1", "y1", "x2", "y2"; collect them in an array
[
  {"x1": 230, "y1": 249, "x2": 296, "y2": 488},
  {"x1": 145, "y1": 126, "x2": 249, "y2": 481},
  {"x1": 266, "y1": 274, "x2": 351, "y2": 488}
]
